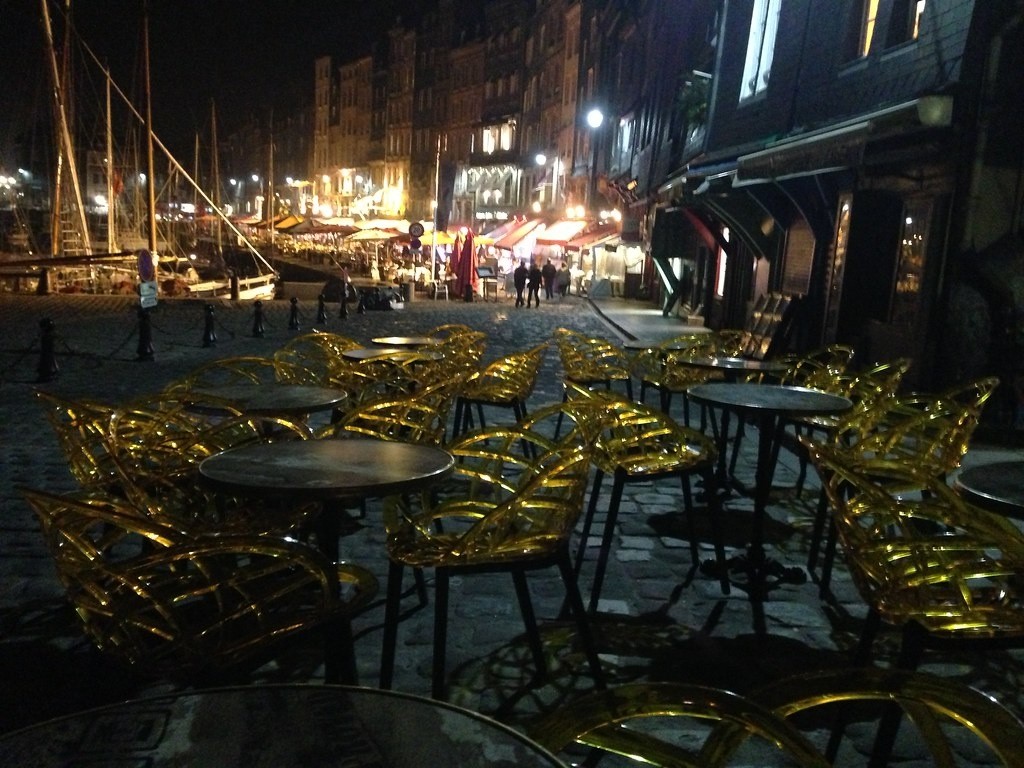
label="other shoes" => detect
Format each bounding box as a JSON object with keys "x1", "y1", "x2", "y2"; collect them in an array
[
  {"x1": 536, "y1": 302, "x2": 539, "y2": 307},
  {"x1": 527, "y1": 304, "x2": 530, "y2": 308},
  {"x1": 520, "y1": 302, "x2": 524, "y2": 307},
  {"x1": 515, "y1": 302, "x2": 518, "y2": 308}
]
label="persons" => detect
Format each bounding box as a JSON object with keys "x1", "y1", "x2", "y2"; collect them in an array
[{"x1": 514, "y1": 258, "x2": 571, "y2": 309}]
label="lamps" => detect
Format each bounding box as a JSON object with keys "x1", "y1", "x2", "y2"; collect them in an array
[{"x1": 915, "y1": 77, "x2": 957, "y2": 130}]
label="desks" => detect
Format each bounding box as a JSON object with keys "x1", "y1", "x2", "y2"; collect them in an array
[
  {"x1": 624, "y1": 342, "x2": 710, "y2": 478},
  {"x1": 341, "y1": 350, "x2": 446, "y2": 395},
  {"x1": 684, "y1": 383, "x2": 855, "y2": 595},
  {"x1": 184, "y1": 384, "x2": 349, "y2": 433},
  {"x1": 678, "y1": 355, "x2": 790, "y2": 502},
  {"x1": 371, "y1": 335, "x2": 444, "y2": 392},
  {"x1": 0, "y1": 683, "x2": 568, "y2": 768},
  {"x1": 957, "y1": 459, "x2": 1024, "y2": 522},
  {"x1": 201, "y1": 440, "x2": 458, "y2": 683}
]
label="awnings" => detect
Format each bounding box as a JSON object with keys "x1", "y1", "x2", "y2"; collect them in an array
[
  {"x1": 536, "y1": 218, "x2": 594, "y2": 247},
  {"x1": 479, "y1": 217, "x2": 546, "y2": 251},
  {"x1": 566, "y1": 226, "x2": 617, "y2": 251}
]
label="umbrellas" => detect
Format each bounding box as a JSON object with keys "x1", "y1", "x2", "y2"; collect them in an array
[{"x1": 449, "y1": 228, "x2": 479, "y2": 298}]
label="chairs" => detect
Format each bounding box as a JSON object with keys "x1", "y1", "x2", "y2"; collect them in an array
[
  {"x1": 433, "y1": 280, "x2": 452, "y2": 301},
  {"x1": 10, "y1": 324, "x2": 1024, "y2": 768}
]
